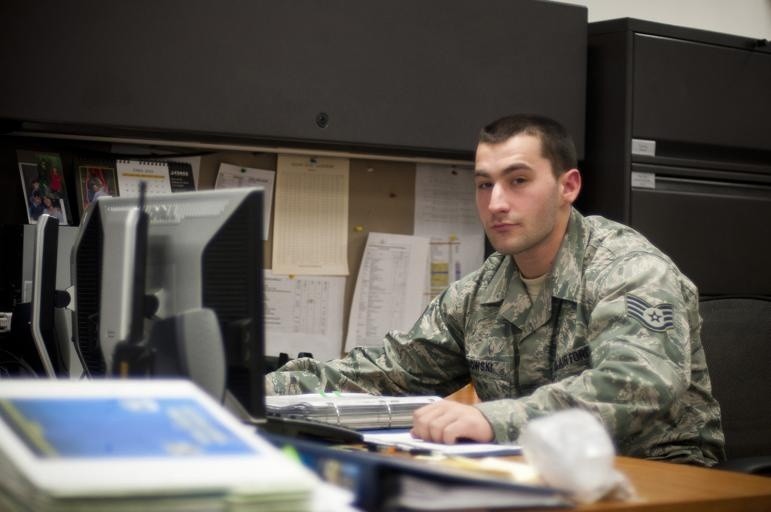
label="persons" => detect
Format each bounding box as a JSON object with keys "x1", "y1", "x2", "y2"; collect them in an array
[
  {"x1": 90, "y1": 175, "x2": 109, "y2": 202},
  {"x1": 265, "y1": 114, "x2": 728, "y2": 472},
  {"x1": 30, "y1": 159, "x2": 64, "y2": 223}
]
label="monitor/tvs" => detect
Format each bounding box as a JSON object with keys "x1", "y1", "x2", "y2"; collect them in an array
[
  {"x1": 23, "y1": 212, "x2": 70, "y2": 383},
  {"x1": 70, "y1": 185, "x2": 268, "y2": 427}
]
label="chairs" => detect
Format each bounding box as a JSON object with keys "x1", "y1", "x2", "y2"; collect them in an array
[{"x1": 695, "y1": 297, "x2": 771, "y2": 479}]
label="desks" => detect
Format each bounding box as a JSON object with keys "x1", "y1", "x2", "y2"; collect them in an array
[{"x1": 456, "y1": 452, "x2": 771, "y2": 510}]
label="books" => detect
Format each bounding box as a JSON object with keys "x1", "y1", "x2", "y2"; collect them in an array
[
  {"x1": 263, "y1": 391, "x2": 442, "y2": 431},
  {"x1": 0, "y1": 375, "x2": 313, "y2": 512}
]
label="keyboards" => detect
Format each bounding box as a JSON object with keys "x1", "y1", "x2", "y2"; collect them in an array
[{"x1": 265, "y1": 410, "x2": 364, "y2": 445}]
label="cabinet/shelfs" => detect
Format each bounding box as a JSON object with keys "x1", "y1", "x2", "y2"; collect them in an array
[{"x1": 590, "y1": 16, "x2": 771, "y2": 295}]
